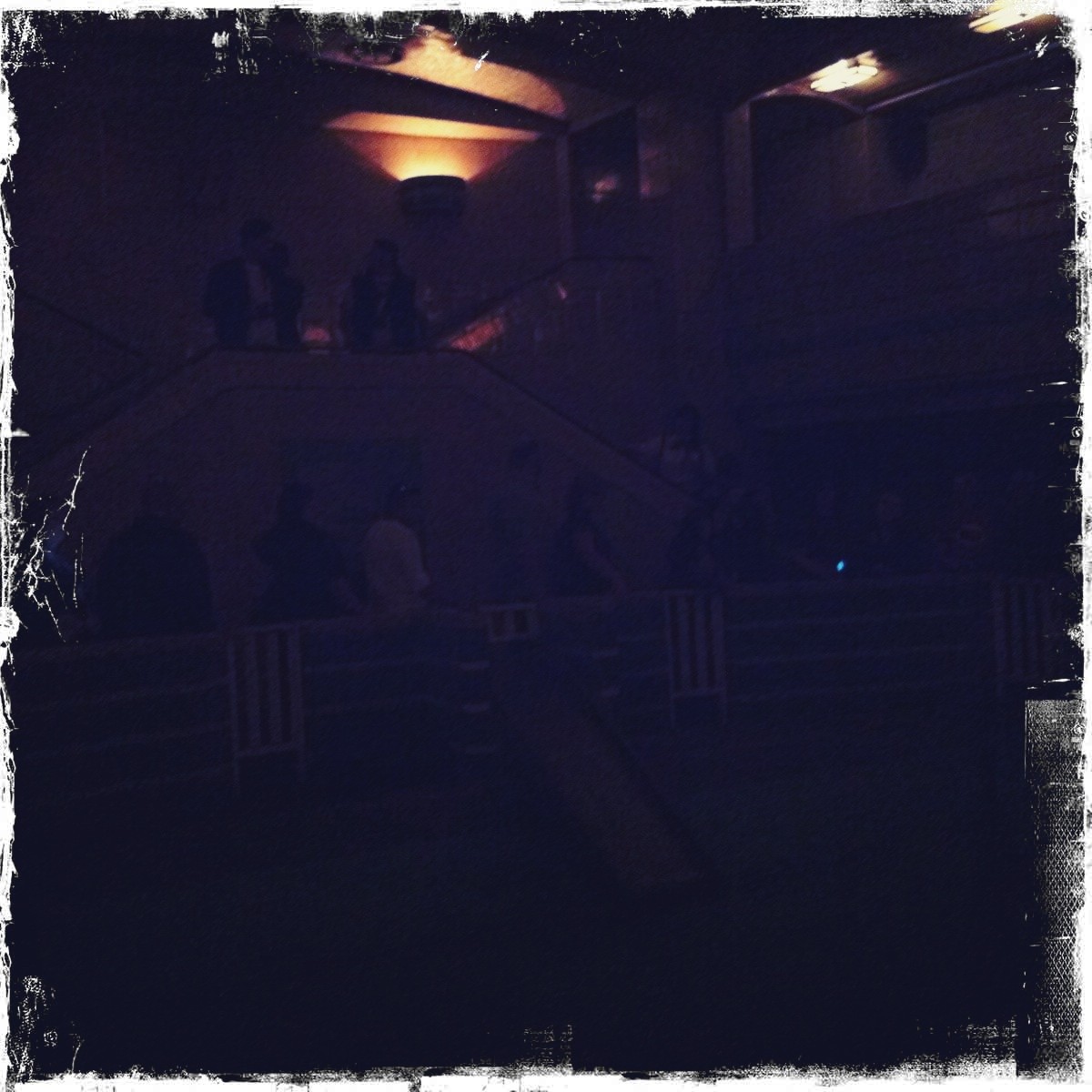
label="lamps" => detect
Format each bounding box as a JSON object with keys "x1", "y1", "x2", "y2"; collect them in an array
[{"x1": 400, "y1": 175, "x2": 467, "y2": 222}]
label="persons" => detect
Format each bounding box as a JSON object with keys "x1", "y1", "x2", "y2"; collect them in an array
[
  {"x1": 360, "y1": 476, "x2": 431, "y2": 611},
  {"x1": 495, "y1": 440, "x2": 559, "y2": 603},
  {"x1": 88, "y1": 480, "x2": 214, "y2": 641},
  {"x1": 652, "y1": 475, "x2": 904, "y2": 590},
  {"x1": 209, "y1": 218, "x2": 299, "y2": 353},
  {"x1": 251, "y1": 483, "x2": 349, "y2": 623},
  {"x1": 556, "y1": 482, "x2": 632, "y2": 606},
  {"x1": 351, "y1": 239, "x2": 427, "y2": 355},
  {"x1": 616, "y1": 403, "x2": 718, "y2": 498},
  {"x1": 16, "y1": 483, "x2": 82, "y2": 651}
]
